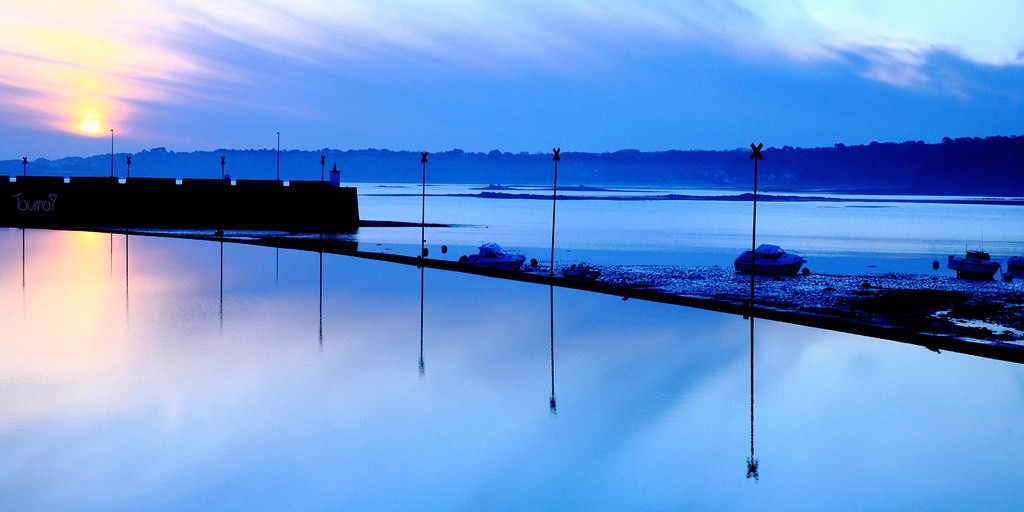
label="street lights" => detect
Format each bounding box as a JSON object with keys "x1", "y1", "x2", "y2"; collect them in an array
[
  {"x1": 275, "y1": 131, "x2": 283, "y2": 181},
  {"x1": 109, "y1": 128, "x2": 114, "y2": 177}
]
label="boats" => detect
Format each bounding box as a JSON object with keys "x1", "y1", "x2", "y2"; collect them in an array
[
  {"x1": 1006, "y1": 251, "x2": 1024, "y2": 271},
  {"x1": 458, "y1": 240, "x2": 528, "y2": 271},
  {"x1": 946, "y1": 222, "x2": 1002, "y2": 280},
  {"x1": 733, "y1": 242, "x2": 809, "y2": 276}
]
